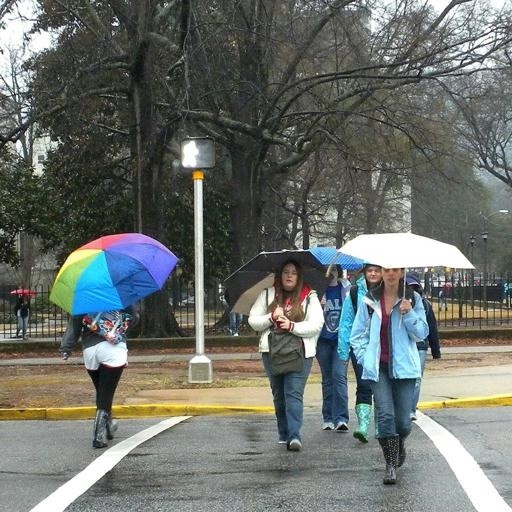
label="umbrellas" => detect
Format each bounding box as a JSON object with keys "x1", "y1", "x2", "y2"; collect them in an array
[
  {"x1": 49, "y1": 233, "x2": 180, "y2": 317},
  {"x1": 310, "y1": 246, "x2": 368, "y2": 270},
  {"x1": 10, "y1": 289, "x2": 38, "y2": 296},
  {"x1": 224, "y1": 250, "x2": 334, "y2": 320},
  {"x1": 337, "y1": 230, "x2": 476, "y2": 315}
]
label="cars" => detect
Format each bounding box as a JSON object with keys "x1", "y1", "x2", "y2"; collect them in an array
[{"x1": 178, "y1": 295, "x2": 194, "y2": 308}]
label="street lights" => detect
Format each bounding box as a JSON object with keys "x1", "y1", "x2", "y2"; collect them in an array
[
  {"x1": 479, "y1": 231, "x2": 488, "y2": 311},
  {"x1": 468, "y1": 234, "x2": 477, "y2": 311},
  {"x1": 479, "y1": 209, "x2": 509, "y2": 229}
]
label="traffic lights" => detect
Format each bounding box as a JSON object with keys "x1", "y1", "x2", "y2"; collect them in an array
[{"x1": 181, "y1": 136, "x2": 219, "y2": 170}]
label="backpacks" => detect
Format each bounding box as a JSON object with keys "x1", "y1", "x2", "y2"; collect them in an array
[{"x1": 267, "y1": 326, "x2": 306, "y2": 377}]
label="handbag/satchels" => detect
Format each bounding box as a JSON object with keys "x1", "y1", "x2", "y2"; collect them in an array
[{"x1": 81, "y1": 309, "x2": 133, "y2": 345}]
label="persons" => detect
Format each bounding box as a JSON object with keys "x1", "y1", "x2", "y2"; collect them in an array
[
  {"x1": 14, "y1": 294, "x2": 30, "y2": 340},
  {"x1": 316, "y1": 264, "x2": 352, "y2": 432},
  {"x1": 337, "y1": 264, "x2": 383, "y2": 443},
  {"x1": 438, "y1": 284, "x2": 449, "y2": 311},
  {"x1": 406, "y1": 273, "x2": 441, "y2": 420},
  {"x1": 59, "y1": 305, "x2": 141, "y2": 447},
  {"x1": 349, "y1": 267, "x2": 430, "y2": 485},
  {"x1": 248, "y1": 259, "x2": 325, "y2": 450},
  {"x1": 225, "y1": 289, "x2": 243, "y2": 336}
]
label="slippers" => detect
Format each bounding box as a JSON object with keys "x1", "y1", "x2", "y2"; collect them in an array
[{"x1": 374, "y1": 407, "x2": 380, "y2": 439}]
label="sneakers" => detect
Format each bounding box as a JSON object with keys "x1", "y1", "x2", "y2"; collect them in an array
[
  {"x1": 409, "y1": 410, "x2": 417, "y2": 420},
  {"x1": 61, "y1": 352, "x2": 71, "y2": 360},
  {"x1": 234, "y1": 333, "x2": 239, "y2": 337},
  {"x1": 278, "y1": 439, "x2": 302, "y2": 452},
  {"x1": 336, "y1": 422, "x2": 349, "y2": 431},
  {"x1": 322, "y1": 422, "x2": 334, "y2": 431}
]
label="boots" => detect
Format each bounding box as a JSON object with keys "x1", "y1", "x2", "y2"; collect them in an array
[
  {"x1": 376, "y1": 430, "x2": 412, "y2": 485},
  {"x1": 106, "y1": 411, "x2": 119, "y2": 441},
  {"x1": 352, "y1": 403, "x2": 372, "y2": 444},
  {"x1": 91, "y1": 409, "x2": 109, "y2": 449}
]
